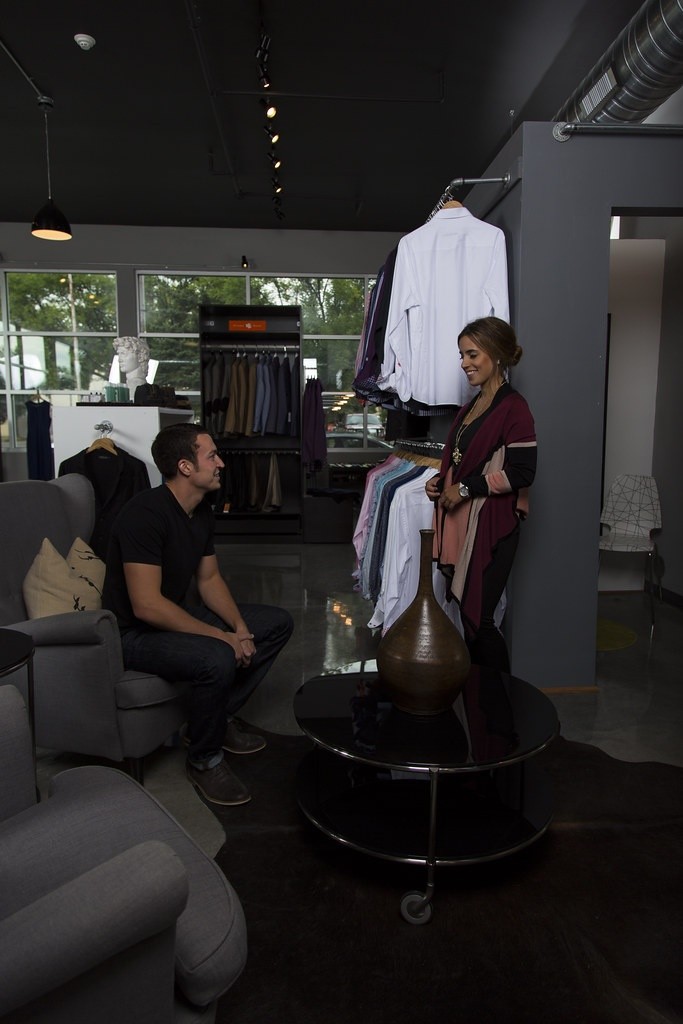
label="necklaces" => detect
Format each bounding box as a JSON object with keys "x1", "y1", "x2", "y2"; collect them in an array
[{"x1": 452, "y1": 380, "x2": 507, "y2": 467}]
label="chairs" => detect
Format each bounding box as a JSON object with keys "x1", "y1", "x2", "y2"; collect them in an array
[{"x1": 598, "y1": 474, "x2": 665, "y2": 630}]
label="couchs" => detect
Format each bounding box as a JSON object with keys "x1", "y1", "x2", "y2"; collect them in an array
[
  {"x1": 0, "y1": 683, "x2": 249, "y2": 1023},
  {"x1": 0, "y1": 473, "x2": 187, "y2": 790}
]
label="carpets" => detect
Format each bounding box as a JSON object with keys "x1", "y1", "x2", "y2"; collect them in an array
[
  {"x1": 597, "y1": 614, "x2": 637, "y2": 653},
  {"x1": 173, "y1": 714, "x2": 683, "y2": 1024}
]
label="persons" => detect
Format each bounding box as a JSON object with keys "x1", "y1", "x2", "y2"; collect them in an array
[
  {"x1": 112, "y1": 336, "x2": 151, "y2": 401},
  {"x1": 423, "y1": 317, "x2": 541, "y2": 679},
  {"x1": 95, "y1": 421, "x2": 295, "y2": 807}
]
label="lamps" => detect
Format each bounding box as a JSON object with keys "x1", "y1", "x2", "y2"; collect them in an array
[
  {"x1": 31, "y1": 95, "x2": 74, "y2": 241},
  {"x1": 271, "y1": 181, "x2": 282, "y2": 193},
  {"x1": 267, "y1": 153, "x2": 281, "y2": 169},
  {"x1": 274, "y1": 208, "x2": 286, "y2": 220},
  {"x1": 262, "y1": 127, "x2": 279, "y2": 143},
  {"x1": 259, "y1": 98, "x2": 276, "y2": 118},
  {"x1": 257, "y1": 62, "x2": 271, "y2": 88}
]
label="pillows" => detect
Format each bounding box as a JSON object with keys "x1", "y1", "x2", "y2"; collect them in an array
[{"x1": 23, "y1": 537, "x2": 107, "y2": 621}]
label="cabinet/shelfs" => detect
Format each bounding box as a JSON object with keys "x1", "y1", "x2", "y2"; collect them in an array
[{"x1": 196, "y1": 303, "x2": 308, "y2": 535}]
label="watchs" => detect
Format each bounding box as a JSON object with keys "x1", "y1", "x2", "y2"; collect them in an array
[{"x1": 457, "y1": 482, "x2": 470, "y2": 500}]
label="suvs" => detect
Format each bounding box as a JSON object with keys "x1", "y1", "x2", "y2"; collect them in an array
[{"x1": 335, "y1": 413, "x2": 386, "y2": 448}]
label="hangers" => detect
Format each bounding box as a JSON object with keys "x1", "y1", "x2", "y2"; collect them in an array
[
  {"x1": 84, "y1": 422, "x2": 118, "y2": 457},
  {"x1": 393, "y1": 437, "x2": 446, "y2": 472},
  {"x1": 424, "y1": 186, "x2": 465, "y2": 225},
  {"x1": 29, "y1": 386, "x2": 53, "y2": 407},
  {"x1": 200, "y1": 342, "x2": 322, "y2": 457}
]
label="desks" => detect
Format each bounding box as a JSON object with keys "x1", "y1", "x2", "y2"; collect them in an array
[
  {"x1": 0, "y1": 626, "x2": 44, "y2": 806},
  {"x1": 77, "y1": 400, "x2": 195, "y2": 488},
  {"x1": 293, "y1": 657, "x2": 564, "y2": 925}
]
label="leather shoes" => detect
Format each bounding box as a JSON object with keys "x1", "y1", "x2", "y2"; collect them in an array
[
  {"x1": 184, "y1": 758, "x2": 251, "y2": 805},
  {"x1": 183, "y1": 720, "x2": 267, "y2": 754}
]
